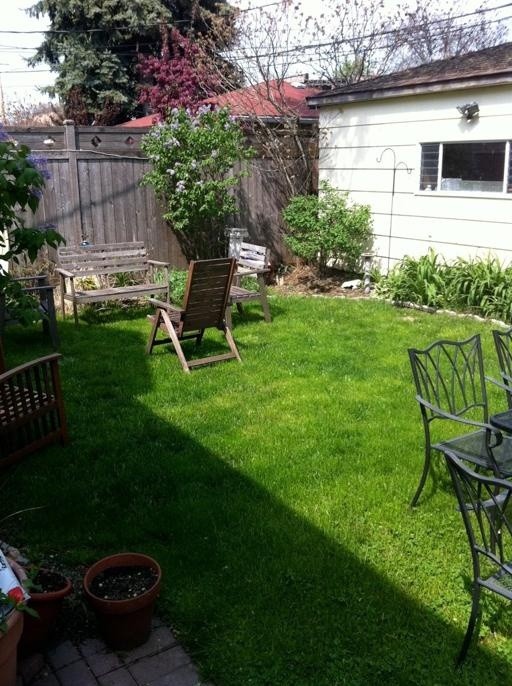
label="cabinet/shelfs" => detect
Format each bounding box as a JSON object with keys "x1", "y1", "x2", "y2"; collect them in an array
[{"x1": 442, "y1": 451, "x2": 512, "y2": 666}]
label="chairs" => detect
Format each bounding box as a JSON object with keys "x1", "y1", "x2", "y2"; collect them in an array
[
  {"x1": 145, "y1": 257, "x2": 244, "y2": 375},
  {"x1": 492, "y1": 329, "x2": 512, "y2": 433},
  {"x1": 0, "y1": 353, "x2": 70, "y2": 468},
  {"x1": 0, "y1": 275, "x2": 60, "y2": 353},
  {"x1": 227, "y1": 242, "x2": 271, "y2": 329},
  {"x1": 408, "y1": 334, "x2": 512, "y2": 508}
]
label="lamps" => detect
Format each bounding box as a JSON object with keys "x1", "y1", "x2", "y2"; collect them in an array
[{"x1": 456, "y1": 101, "x2": 480, "y2": 119}]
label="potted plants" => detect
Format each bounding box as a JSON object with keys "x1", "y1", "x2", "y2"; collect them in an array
[{"x1": 0, "y1": 543, "x2": 33, "y2": 686}]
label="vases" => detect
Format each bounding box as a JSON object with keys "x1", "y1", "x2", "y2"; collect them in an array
[
  {"x1": 83, "y1": 553, "x2": 162, "y2": 649},
  {"x1": 19, "y1": 568, "x2": 73, "y2": 651}
]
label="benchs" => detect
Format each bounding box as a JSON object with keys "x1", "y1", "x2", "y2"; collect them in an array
[{"x1": 54, "y1": 241, "x2": 172, "y2": 325}]
